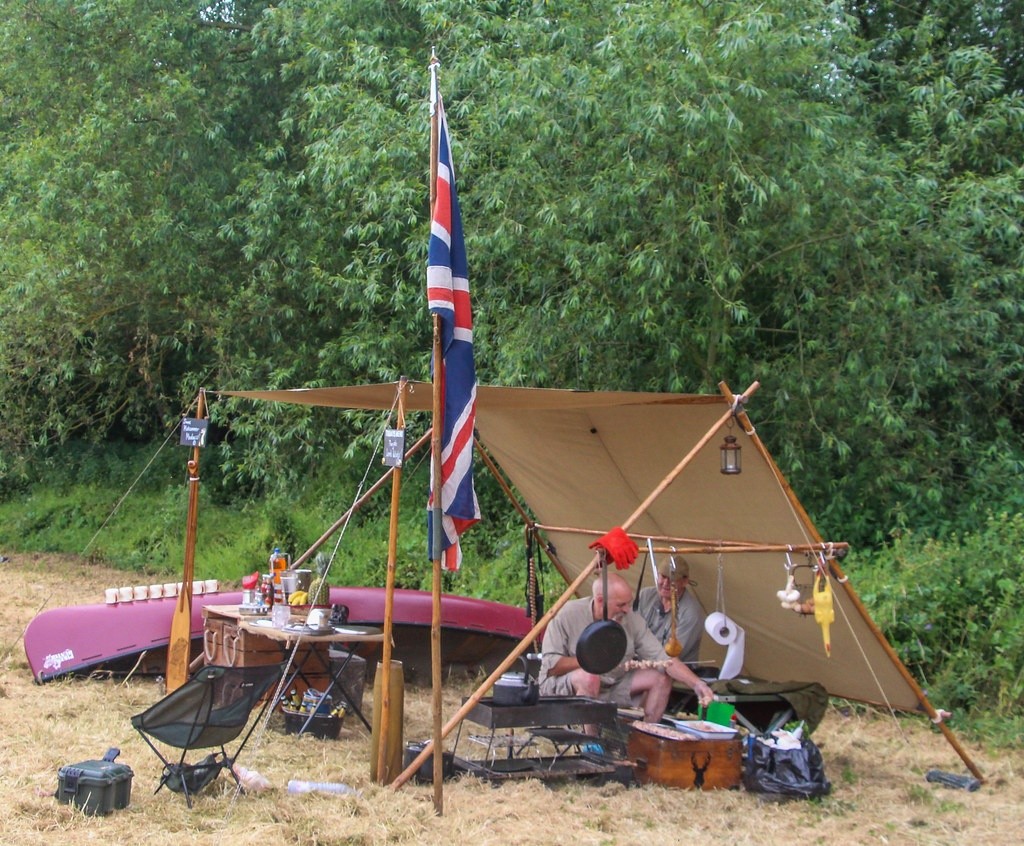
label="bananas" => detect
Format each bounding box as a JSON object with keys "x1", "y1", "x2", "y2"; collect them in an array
[{"x1": 288, "y1": 590, "x2": 309, "y2": 605}]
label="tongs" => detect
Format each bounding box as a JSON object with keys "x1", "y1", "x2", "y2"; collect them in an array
[{"x1": 633, "y1": 538, "x2": 661, "y2": 611}]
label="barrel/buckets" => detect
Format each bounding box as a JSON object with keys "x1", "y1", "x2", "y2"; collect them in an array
[{"x1": 282, "y1": 707, "x2": 344, "y2": 739}]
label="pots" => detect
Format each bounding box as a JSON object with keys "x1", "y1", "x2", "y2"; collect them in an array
[{"x1": 576, "y1": 558, "x2": 627, "y2": 674}]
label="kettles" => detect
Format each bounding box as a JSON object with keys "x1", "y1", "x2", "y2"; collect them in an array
[
  {"x1": 493, "y1": 656, "x2": 540, "y2": 706},
  {"x1": 403, "y1": 741, "x2": 453, "y2": 781}
]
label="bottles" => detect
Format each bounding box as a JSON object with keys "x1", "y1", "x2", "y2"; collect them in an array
[
  {"x1": 281, "y1": 690, "x2": 347, "y2": 717},
  {"x1": 269, "y1": 548, "x2": 286, "y2": 582},
  {"x1": 261, "y1": 576, "x2": 267, "y2": 595},
  {"x1": 265, "y1": 577, "x2": 274, "y2": 610}
]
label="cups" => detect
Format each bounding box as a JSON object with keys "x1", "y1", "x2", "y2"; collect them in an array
[
  {"x1": 243, "y1": 589, "x2": 255, "y2": 604},
  {"x1": 280, "y1": 571, "x2": 299, "y2": 605}
]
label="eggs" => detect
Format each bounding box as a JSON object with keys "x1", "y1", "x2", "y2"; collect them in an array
[{"x1": 781, "y1": 598, "x2": 815, "y2": 614}]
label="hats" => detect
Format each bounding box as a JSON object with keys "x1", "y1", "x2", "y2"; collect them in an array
[{"x1": 658, "y1": 555, "x2": 688, "y2": 579}]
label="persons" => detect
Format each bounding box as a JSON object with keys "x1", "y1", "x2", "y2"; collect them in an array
[
  {"x1": 631, "y1": 554, "x2": 702, "y2": 669},
  {"x1": 540, "y1": 572, "x2": 713, "y2": 756}
]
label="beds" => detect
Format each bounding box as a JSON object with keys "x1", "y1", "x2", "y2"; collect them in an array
[{"x1": 526, "y1": 653, "x2": 827, "y2": 759}]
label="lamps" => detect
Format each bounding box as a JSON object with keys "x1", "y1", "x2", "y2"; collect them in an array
[{"x1": 719, "y1": 415, "x2": 740, "y2": 475}]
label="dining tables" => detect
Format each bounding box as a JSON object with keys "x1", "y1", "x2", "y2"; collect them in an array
[{"x1": 238, "y1": 620, "x2": 394, "y2": 740}]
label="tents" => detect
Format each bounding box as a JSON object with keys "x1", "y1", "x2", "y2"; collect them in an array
[{"x1": 158, "y1": 374, "x2": 989, "y2": 791}]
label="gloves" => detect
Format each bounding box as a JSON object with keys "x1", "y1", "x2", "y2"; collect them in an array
[{"x1": 589, "y1": 526, "x2": 639, "y2": 570}]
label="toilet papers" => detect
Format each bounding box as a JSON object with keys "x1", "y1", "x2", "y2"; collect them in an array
[{"x1": 705, "y1": 613, "x2": 746, "y2": 683}]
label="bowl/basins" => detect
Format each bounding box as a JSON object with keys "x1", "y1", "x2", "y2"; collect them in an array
[{"x1": 289, "y1": 604, "x2": 332, "y2": 616}]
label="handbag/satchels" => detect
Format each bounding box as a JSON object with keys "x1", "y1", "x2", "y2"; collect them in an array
[{"x1": 745, "y1": 729, "x2": 831, "y2": 799}]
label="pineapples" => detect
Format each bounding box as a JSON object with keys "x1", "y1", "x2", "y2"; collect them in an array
[{"x1": 307, "y1": 554, "x2": 330, "y2": 606}]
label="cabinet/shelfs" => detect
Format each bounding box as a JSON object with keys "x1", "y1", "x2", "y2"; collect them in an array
[{"x1": 200, "y1": 604, "x2": 329, "y2": 708}]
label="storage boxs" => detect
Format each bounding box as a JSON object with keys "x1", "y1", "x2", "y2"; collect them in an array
[
  {"x1": 53, "y1": 748, "x2": 134, "y2": 817},
  {"x1": 625, "y1": 727, "x2": 742, "y2": 793}
]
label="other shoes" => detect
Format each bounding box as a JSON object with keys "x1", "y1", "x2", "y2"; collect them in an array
[{"x1": 584, "y1": 743, "x2": 606, "y2": 754}]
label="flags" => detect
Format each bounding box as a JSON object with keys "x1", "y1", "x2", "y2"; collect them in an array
[{"x1": 426, "y1": 99, "x2": 483, "y2": 574}]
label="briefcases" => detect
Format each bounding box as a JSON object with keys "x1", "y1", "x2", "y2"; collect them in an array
[{"x1": 57, "y1": 748, "x2": 135, "y2": 816}]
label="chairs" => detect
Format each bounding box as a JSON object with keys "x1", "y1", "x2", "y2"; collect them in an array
[{"x1": 130, "y1": 660, "x2": 287, "y2": 809}]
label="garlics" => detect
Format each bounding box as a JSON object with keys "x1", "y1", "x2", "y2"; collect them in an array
[{"x1": 776, "y1": 589, "x2": 800, "y2": 602}]
